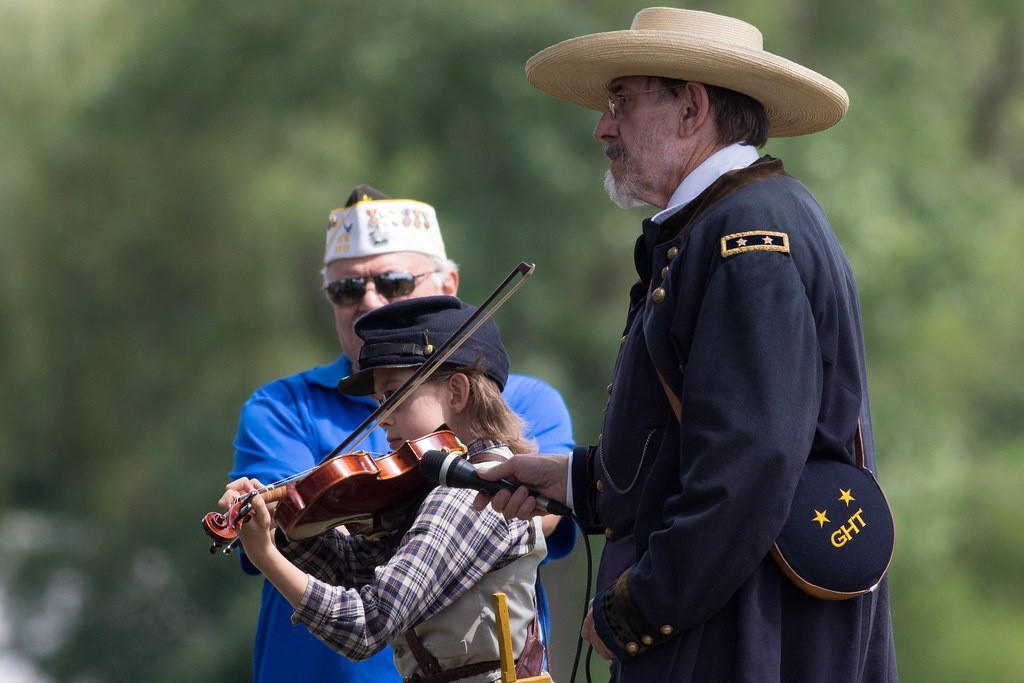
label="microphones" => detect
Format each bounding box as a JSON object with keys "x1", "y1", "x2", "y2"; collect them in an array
[{"x1": 420, "y1": 449, "x2": 571, "y2": 516}]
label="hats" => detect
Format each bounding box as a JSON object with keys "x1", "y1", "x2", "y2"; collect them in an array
[
  {"x1": 337, "y1": 296, "x2": 510, "y2": 396},
  {"x1": 525, "y1": 6, "x2": 849, "y2": 137},
  {"x1": 323, "y1": 183, "x2": 446, "y2": 263}
]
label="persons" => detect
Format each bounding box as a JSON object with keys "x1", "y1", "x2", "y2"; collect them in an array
[
  {"x1": 473, "y1": 6, "x2": 897, "y2": 683},
  {"x1": 229, "y1": 187, "x2": 577, "y2": 683},
  {"x1": 217, "y1": 295, "x2": 550, "y2": 683}
]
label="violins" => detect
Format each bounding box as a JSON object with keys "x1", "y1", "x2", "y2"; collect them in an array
[{"x1": 200, "y1": 423, "x2": 469, "y2": 555}]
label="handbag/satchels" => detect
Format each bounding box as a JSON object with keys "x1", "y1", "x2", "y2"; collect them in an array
[{"x1": 767, "y1": 460, "x2": 896, "y2": 600}]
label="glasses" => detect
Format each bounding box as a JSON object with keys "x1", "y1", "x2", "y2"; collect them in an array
[
  {"x1": 323, "y1": 269, "x2": 450, "y2": 308},
  {"x1": 607, "y1": 83, "x2": 696, "y2": 119}
]
